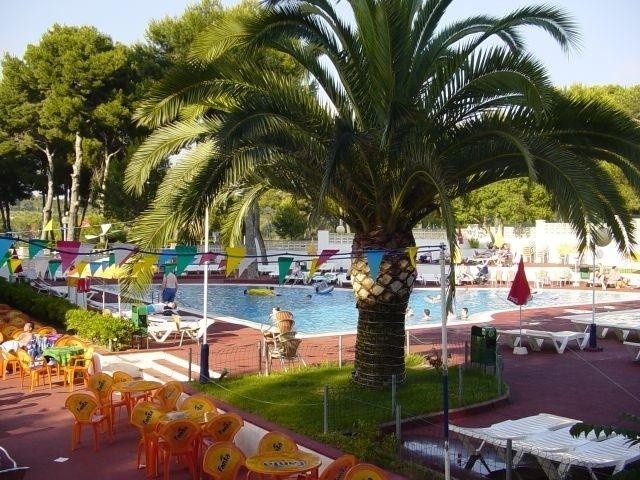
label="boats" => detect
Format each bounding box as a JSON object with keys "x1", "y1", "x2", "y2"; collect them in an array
[{"x1": 88, "y1": 241, "x2": 115, "y2": 258}]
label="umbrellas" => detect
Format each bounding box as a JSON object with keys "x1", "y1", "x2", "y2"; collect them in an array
[
  {"x1": 450, "y1": 240, "x2": 461, "y2": 271},
  {"x1": 507, "y1": 256, "x2": 533, "y2": 348},
  {"x1": 493, "y1": 225, "x2": 506, "y2": 264}
]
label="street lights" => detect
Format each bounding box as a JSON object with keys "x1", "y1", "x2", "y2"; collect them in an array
[
  {"x1": 61, "y1": 214, "x2": 70, "y2": 242},
  {"x1": 336, "y1": 207, "x2": 345, "y2": 244},
  {"x1": 581, "y1": 226, "x2": 616, "y2": 353}
]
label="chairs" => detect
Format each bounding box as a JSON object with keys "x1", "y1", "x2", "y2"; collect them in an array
[
  {"x1": 497, "y1": 306, "x2": 640, "y2": 365},
  {"x1": 52, "y1": 285, "x2": 217, "y2": 348},
  {"x1": 447, "y1": 404, "x2": 640, "y2": 480},
  {"x1": 1, "y1": 304, "x2": 96, "y2": 395},
  {"x1": 403, "y1": 239, "x2": 635, "y2": 271}
]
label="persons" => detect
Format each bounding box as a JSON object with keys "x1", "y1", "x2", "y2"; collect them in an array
[
  {"x1": 420, "y1": 309, "x2": 432, "y2": 320},
  {"x1": 406, "y1": 307, "x2": 415, "y2": 320},
  {"x1": 290, "y1": 261, "x2": 301, "y2": 277},
  {"x1": 159, "y1": 271, "x2": 178, "y2": 302},
  {"x1": 270, "y1": 306, "x2": 280, "y2": 324},
  {"x1": 596, "y1": 263, "x2": 621, "y2": 292},
  {"x1": 426, "y1": 294, "x2": 440, "y2": 304},
  {"x1": 4, "y1": 320, "x2": 34, "y2": 351},
  {"x1": 459, "y1": 308, "x2": 469, "y2": 319}
]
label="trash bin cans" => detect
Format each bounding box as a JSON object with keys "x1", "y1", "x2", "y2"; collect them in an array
[
  {"x1": 470, "y1": 325, "x2": 496, "y2": 366},
  {"x1": 131, "y1": 303, "x2": 147, "y2": 334}
]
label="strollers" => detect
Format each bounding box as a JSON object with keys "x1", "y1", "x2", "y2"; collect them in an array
[{"x1": 472, "y1": 259, "x2": 493, "y2": 285}]
